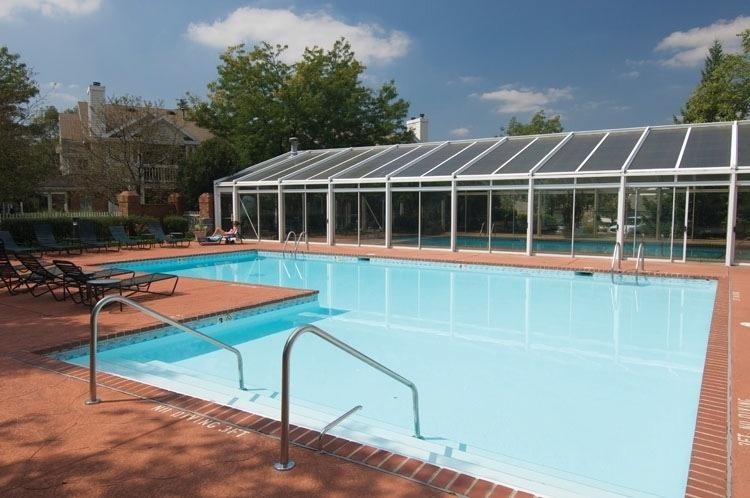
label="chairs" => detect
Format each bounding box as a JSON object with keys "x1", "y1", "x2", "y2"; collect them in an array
[
  {"x1": 198, "y1": 225, "x2": 243, "y2": 244},
  {"x1": 0, "y1": 220, "x2": 190, "y2": 311}
]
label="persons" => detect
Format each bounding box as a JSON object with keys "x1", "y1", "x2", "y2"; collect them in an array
[{"x1": 211, "y1": 220, "x2": 241, "y2": 238}]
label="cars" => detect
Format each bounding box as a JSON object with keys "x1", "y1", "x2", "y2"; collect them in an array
[{"x1": 609, "y1": 216, "x2": 643, "y2": 235}]
